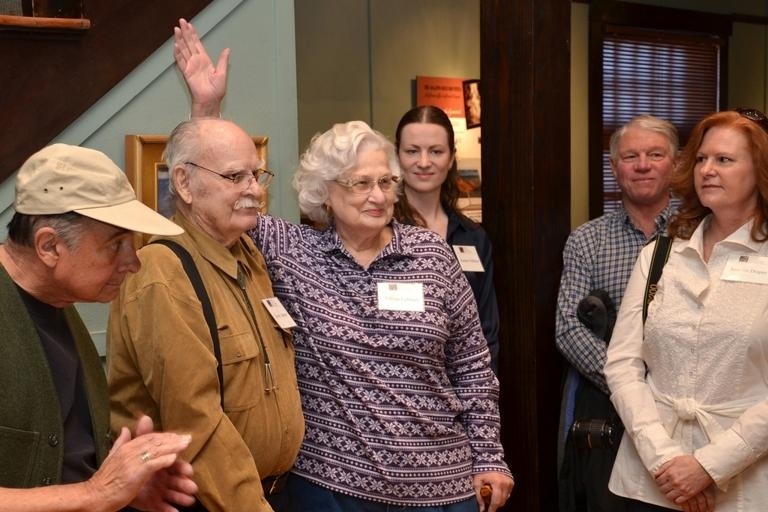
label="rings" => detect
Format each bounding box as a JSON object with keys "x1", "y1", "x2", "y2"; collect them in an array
[{"x1": 507, "y1": 492, "x2": 512, "y2": 499}]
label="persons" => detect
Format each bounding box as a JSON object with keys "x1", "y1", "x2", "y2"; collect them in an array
[
  {"x1": 393, "y1": 104, "x2": 503, "y2": 377},
  {"x1": 0, "y1": 141, "x2": 202, "y2": 511},
  {"x1": 171, "y1": 17, "x2": 517, "y2": 511},
  {"x1": 138, "y1": 449, "x2": 155, "y2": 462},
  {"x1": 103, "y1": 115, "x2": 310, "y2": 511},
  {"x1": 552, "y1": 113, "x2": 686, "y2": 512},
  {"x1": 603, "y1": 109, "x2": 768, "y2": 511}
]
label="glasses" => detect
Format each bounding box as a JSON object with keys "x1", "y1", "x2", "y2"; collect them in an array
[
  {"x1": 334, "y1": 176, "x2": 395, "y2": 194},
  {"x1": 184, "y1": 161, "x2": 275, "y2": 193},
  {"x1": 735, "y1": 107, "x2": 768, "y2": 131}
]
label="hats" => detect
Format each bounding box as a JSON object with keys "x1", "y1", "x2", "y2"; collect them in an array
[{"x1": 14, "y1": 144, "x2": 185, "y2": 237}]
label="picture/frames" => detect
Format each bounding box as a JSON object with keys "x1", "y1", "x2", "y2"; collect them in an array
[{"x1": 124, "y1": 132, "x2": 269, "y2": 250}]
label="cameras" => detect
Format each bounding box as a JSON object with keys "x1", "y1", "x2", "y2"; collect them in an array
[{"x1": 568, "y1": 409, "x2": 623, "y2": 457}]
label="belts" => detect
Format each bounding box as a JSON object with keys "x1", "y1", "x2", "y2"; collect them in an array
[{"x1": 262, "y1": 470, "x2": 290, "y2": 495}]
